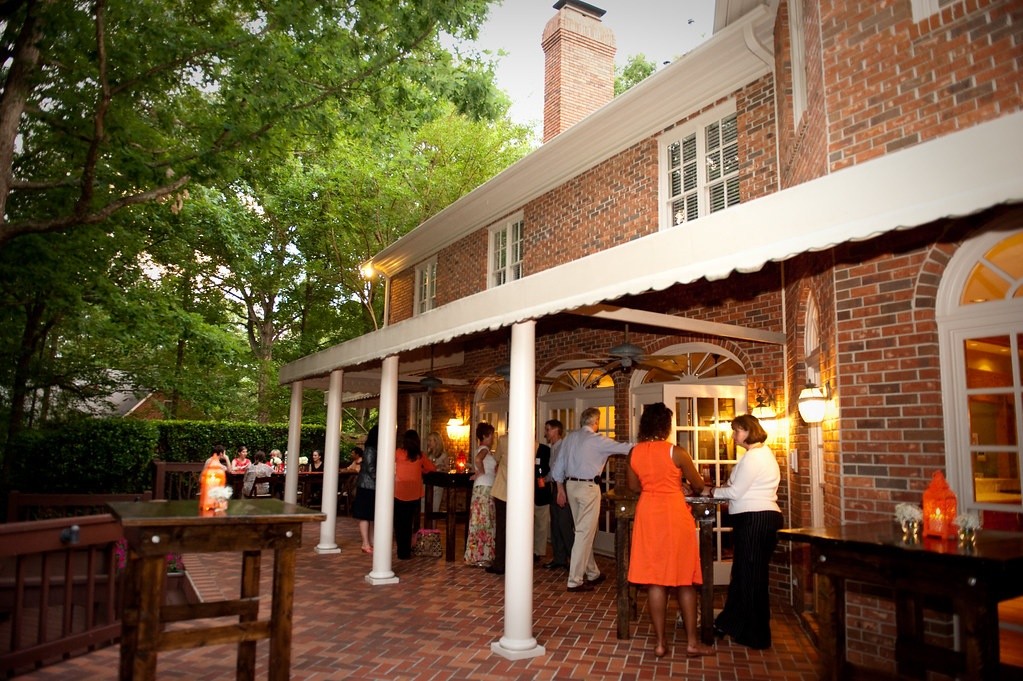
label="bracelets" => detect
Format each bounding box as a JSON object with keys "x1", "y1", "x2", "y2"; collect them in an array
[{"x1": 710, "y1": 487, "x2": 715, "y2": 495}]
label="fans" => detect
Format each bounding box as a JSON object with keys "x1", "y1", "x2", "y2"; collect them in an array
[
  {"x1": 465, "y1": 337, "x2": 557, "y2": 383},
  {"x1": 398, "y1": 346, "x2": 473, "y2": 392},
  {"x1": 557, "y1": 322, "x2": 686, "y2": 374}
]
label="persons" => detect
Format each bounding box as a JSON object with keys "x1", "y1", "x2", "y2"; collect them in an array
[
  {"x1": 702, "y1": 415, "x2": 782, "y2": 649},
  {"x1": 552, "y1": 408, "x2": 636, "y2": 592},
  {"x1": 629, "y1": 402, "x2": 718, "y2": 657},
  {"x1": 204, "y1": 420, "x2": 577, "y2": 575}
]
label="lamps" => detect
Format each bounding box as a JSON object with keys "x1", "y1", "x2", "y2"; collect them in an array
[
  {"x1": 923, "y1": 471, "x2": 959, "y2": 539},
  {"x1": 796, "y1": 378, "x2": 832, "y2": 423},
  {"x1": 447, "y1": 409, "x2": 463, "y2": 439},
  {"x1": 456, "y1": 448, "x2": 467, "y2": 471},
  {"x1": 750, "y1": 386, "x2": 777, "y2": 430}
]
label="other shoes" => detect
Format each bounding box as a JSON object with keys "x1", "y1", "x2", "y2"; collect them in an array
[
  {"x1": 485, "y1": 566, "x2": 504, "y2": 575},
  {"x1": 687, "y1": 644, "x2": 716, "y2": 656},
  {"x1": 566, "y1": 583, "x2": 593, "y2": 592},
  {"x1": 542, "y1": 560, "x2": 559, "y2": 568},
  {"x1": 589, "y1": 573, "x2": 605, "y2": 585},
  {"x1": 654, "y1": 644, "x2": 668, "y2": 657},
  {"x1": 361, "y1": 544, "x2": 374, "y2": 553}
]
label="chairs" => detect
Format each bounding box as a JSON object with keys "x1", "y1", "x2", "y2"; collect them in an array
[{"x1": 244, "y1": 476, "x2": 272, "y2": 499}]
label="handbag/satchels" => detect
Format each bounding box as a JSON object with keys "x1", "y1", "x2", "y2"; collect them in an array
[{"x1": 413, "y1": 529, "x2": 443, "y2": 557}]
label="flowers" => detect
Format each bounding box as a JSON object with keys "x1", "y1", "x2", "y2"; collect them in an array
[{"x1": 114, "y1": 539, "x2": 186, "y2": 574}]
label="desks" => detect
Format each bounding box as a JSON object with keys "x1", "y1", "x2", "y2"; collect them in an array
[
  {"x1": 413, "y1": 470, "x2": 475, "y2": 561},
  {"x1": 974, "y1": 476, "x2": 1021, "y2": 493},
  {"x1": 226, "y1": 471, "x2": 247, "y2": 500},
  {"x1": 105, "y1": 496, "x2": 327, "y2": 681},
  {"x1": 775, "y1": 519, "x2": 1023, "y2": 681},
  {"x1": 269, "y1": 470, "x2": 360, "y2": 514},
  {"x1": 604, "y1": 487, "x2": 728, "y2": 645}
]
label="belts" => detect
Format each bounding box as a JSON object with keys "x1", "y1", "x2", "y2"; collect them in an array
[{"x1": 565, "y1": 477, "x2": 593, "y2": 482}]
